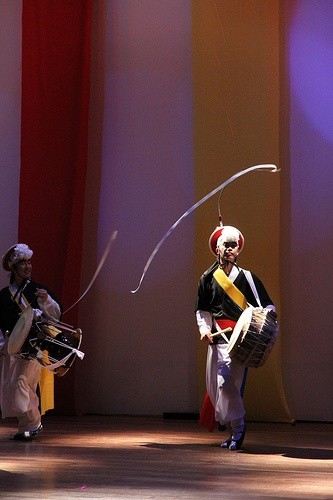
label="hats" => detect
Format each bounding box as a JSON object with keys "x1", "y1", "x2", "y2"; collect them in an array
[
  {"x1": 208, "y1": 225, "x2": 245, "y2": 258},
  {"x1": 0, "y1": 243, "x2": 33, "y2": 272}
]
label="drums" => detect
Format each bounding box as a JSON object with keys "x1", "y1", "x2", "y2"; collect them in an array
[
  {"x1": 7, "y1": 306, "x2": 83, "y2": 377},
  {"x1": 227, "y1": 307, "x2": 281, "y2": 369}
]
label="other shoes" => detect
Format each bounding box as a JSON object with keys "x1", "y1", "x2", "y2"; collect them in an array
[
  {"x1": 229, "y1": 421, "x2": 247, "y2": 450},
  {"x1": 220, "y1": 434, "x2": 233, "y2": 448},
  {"x1": 10, "y1": 424, "x2": 43, "y2": 441}
]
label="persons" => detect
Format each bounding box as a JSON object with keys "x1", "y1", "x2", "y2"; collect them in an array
[
  {"x1": 194, "y1": 225, "x2": 277, "y2": 450},
  {"x1": 0, "y1": 243, "x2": 63, "y2": 441}
]
